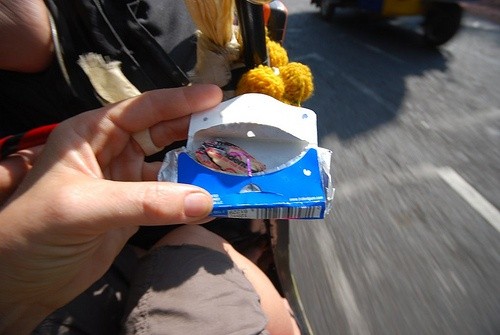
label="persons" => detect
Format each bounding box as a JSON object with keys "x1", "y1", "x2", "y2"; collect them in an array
[
  {"x1": 0, "y1": 85, "x2": 295, "y2": 335},
  {"x1": 1, "y1": 1, "x2": 278, "y2": 266}
]
